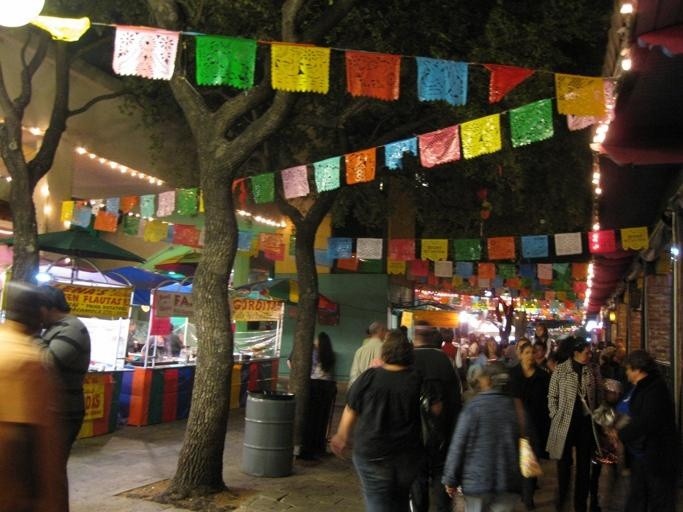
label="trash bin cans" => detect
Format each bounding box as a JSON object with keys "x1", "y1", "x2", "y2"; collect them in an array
[
  {"x1": 240, "y1": 389, "x2": 296, "y2": 477},
  {"x1": 307, "y1": 379, "x2": 337, "y2": 441}
]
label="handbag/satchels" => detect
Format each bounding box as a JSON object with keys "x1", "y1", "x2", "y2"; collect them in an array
[
  {"x1": 418, "y1": 372, "x2": 451, "y2": 452},
  {"x1": 519, "y1": 436, "x2": 544, "y2": 478}
]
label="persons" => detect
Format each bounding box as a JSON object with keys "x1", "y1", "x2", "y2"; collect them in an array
[
  {"x1": 285, "y1": 350, "x2": 293, "y2": 371},
  {"x1": 138, "y1": 332, "x2": 169, "y2": 359},
  {"x1": 0, "y1": 288, "x2": 69, "y2": 510},
  {"x1": 31, "y1": 283, "x2": 92, "y2": 512},
  {"x1": 123, "y1": 321, "x2": 139, "y2": 365},
  {"x1": 307, "y1": 332, "x2": 337, "y2": 453},
  {"x1": 161, "y1": 323, "x2": 185, "y2": 358}
]
